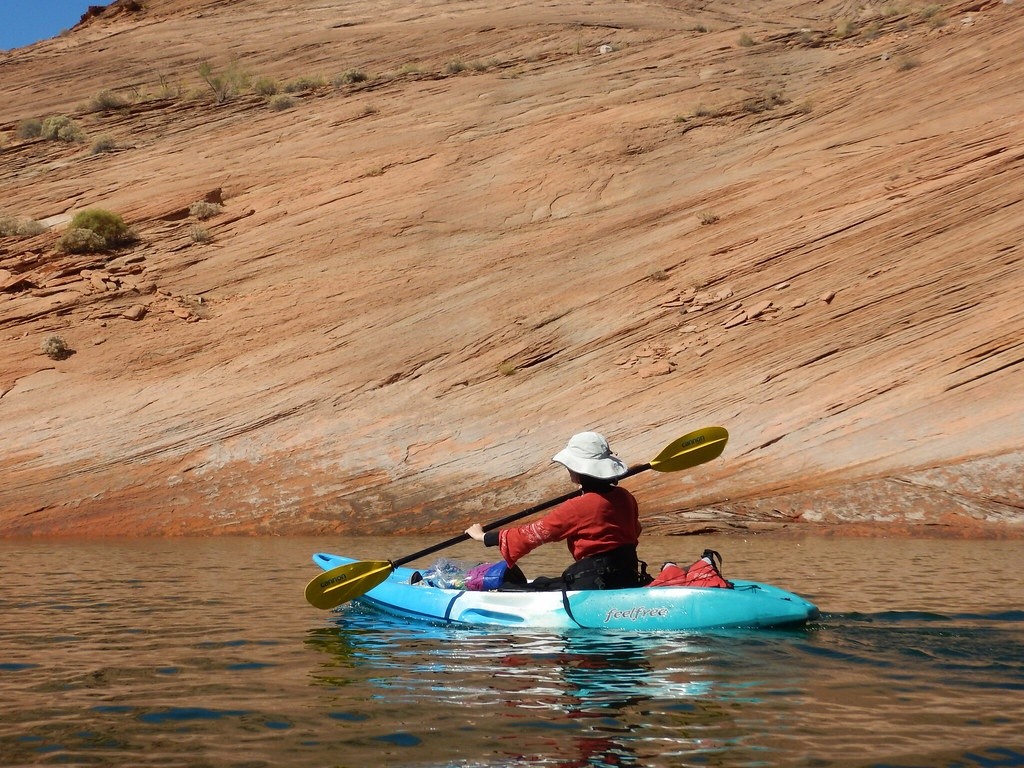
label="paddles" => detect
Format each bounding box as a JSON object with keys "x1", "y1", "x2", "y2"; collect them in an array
[{"x1": 304, "y1": 426, "x2": 729, "y2": 611}]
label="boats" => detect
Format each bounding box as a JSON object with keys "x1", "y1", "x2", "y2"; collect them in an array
[{"x1": 308, "y1": 549, "x2": 820, "y2": 636}]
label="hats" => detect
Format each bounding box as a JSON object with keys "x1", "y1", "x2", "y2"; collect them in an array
[{"x1": 553, "y1": 431, "x2": 628, "y2": 481}]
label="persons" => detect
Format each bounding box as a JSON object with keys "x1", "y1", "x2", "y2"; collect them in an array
[{"x1": 409, "y1": 431, "x2": 643, "y2": 592}]
